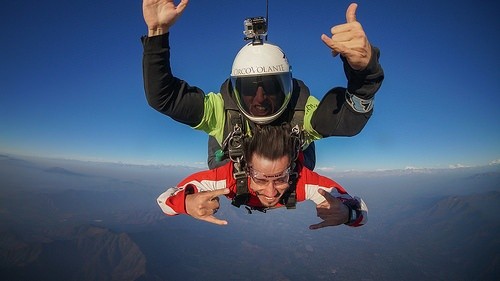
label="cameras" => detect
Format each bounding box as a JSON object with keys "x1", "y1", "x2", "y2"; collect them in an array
[{"x1": 243, "y1": 16, "x2": 269, "y2": 38}]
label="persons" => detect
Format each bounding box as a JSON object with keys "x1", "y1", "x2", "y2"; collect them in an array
[
  {"x1": 140, "y1": 1, "x2": 384, "y2": 172},
  {"x1": 157, "y1": 123, "x2": 370, "y2": 233}
]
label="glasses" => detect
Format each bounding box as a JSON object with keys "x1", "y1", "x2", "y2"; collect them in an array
[
  {"x1": 247, "y1": 171, "x2": 292, "y2": 187},
  {"x1": 236, "y1": 80, "x2": 286, "y2": 97}
]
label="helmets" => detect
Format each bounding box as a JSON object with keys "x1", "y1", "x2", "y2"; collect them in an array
[{"x1": 231, "y1": 41, "x2": 296, "y2": 126}]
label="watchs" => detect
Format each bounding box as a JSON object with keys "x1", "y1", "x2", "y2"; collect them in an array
[{"x1": 344, "y1": 202, "x2": 358, "y2": 226}]
